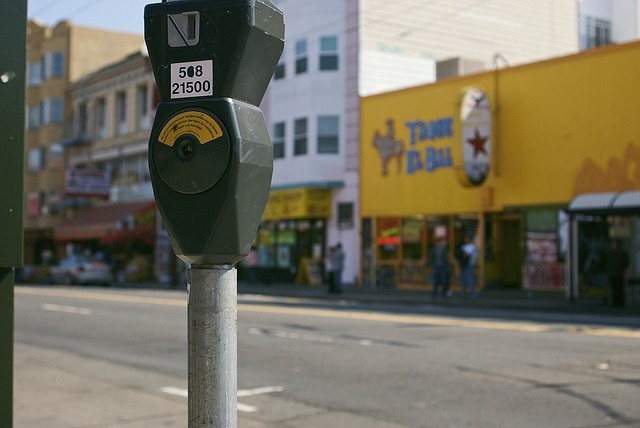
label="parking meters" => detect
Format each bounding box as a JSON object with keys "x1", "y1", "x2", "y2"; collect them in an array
[{"x1": 142, "y1": 0, "x2": 286, "y2": 428}]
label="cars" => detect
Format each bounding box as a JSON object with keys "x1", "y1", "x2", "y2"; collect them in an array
[{"x1": 48, "y1": 253, "x2": 113, "y2": 285}]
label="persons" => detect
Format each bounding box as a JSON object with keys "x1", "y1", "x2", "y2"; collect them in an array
[
  {"x1": 243, "y1": 247, "x2": 258, "y2": 287},
  {"x1": 459, "y1": 235, "x2": 477, "y2": 297},
  {"x1": 326, "y1": 242, "x2": 345, "y2": 295},
  {"x1": 431, "y1": 235, "x2": 453, "y2": 298}
]
